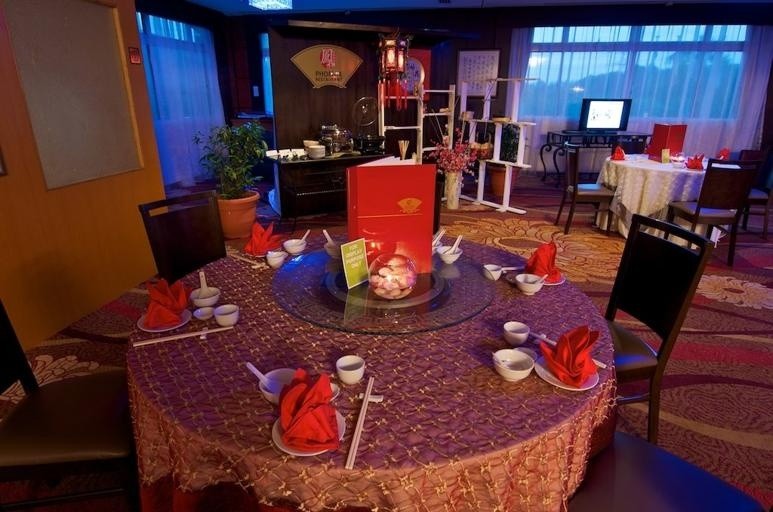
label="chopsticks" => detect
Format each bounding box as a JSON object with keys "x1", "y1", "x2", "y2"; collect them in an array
[
  {"x1": 132, "y1": 324, "x2": 233, "y2": 347},
  {"x1": 343, "y1": 376, "x2": 374, "y2": 472},
  {"x1": 502, "y1": 265, "x2": 526, "y2": 270},
  {"x1": 530, "y1": 332, "x2": 608, "y2": 369},
  {"x1": 231, "y1": 253, "x2": 268, "y2": 269}
]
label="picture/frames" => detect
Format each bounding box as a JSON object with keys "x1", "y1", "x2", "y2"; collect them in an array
[{"x1": 454, "y1": 47, "x2": 501, "y2": 98}]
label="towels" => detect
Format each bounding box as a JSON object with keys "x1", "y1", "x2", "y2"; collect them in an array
[
  {"x1": 526, "y1": 238, "x2": 563, "y2": 280},
  {"x1": 534, "y1": 326, "x2": 602, "y2": 389},
  {"x1": 276, "y1": 363, "x2": 342, "y2": 452},
  {"x1": 139, "y1": 274, "x2": 188, "y2": 329},
  {"x1": 244, "y1": 220, "x2": 284, "y2": 255}
]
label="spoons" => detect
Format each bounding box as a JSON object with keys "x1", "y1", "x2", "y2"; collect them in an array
[
  {"x1": 321, "y1": 229, "x2": 336, "y2": 248},
  {"x1": 491, "y1": 352, "x2": 521, "y2": 370},
  {"x1": 295, "y1": 229, "x2": 310, "y2": 246},
  {"x1": 246, "y1": 362, "x2": 277, "y2": 394},
  {"x1": 431, "y1": 227, "x2": 446, "y2": 247},
  {"x1": 444, "y1": 234, "x2": 462, "y2": 255},
  {"x1": 197, "y1": 272, "x2": 212, "y2": 299}
]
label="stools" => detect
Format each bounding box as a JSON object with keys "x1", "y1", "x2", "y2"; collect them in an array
[{"x1": 563, "y1": 430, "x2": 763, "y2": 510}]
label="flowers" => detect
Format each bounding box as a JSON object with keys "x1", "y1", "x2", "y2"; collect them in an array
[{"x1": 410, "y1": 86, "x2": 492, "y2": 189}]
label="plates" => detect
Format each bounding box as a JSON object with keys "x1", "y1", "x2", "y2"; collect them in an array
[
  {"x1": 193, "y1": 307, "x2": 214, "y2": 320},
  {"x1": 329, "y1": 383, "x2": 340, "y2": 404},
  {"x1": 535, "y1": 355, "x2": 598, "y2": 391},
  {"x1": 514, "y1": 347, "x2": 538, "y2": 361},
  {"x1": 505, "y1": 273, "x2": 518, "y2": 285},
  {"x1": 542, "y1": 274, "x2": 566, "y2": 286},
  {"x1": 272, "y1": 410, "x2": 345, "y2": 456},
  {"x1": 136, "y1": 309, "x2": 193, "y2": 332}
]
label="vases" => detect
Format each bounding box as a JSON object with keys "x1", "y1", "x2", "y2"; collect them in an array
[{"x1": 445, "y1": 171, "x2": 462, "y2": 209}]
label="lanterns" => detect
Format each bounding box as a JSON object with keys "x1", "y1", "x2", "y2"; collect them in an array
[{"x1": 376, "y1": 36, "x2": 409, "y2": 112}]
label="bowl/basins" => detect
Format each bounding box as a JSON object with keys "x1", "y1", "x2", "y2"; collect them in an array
[
  {"x1": 283, "y1": 239, "x2": 306, "y2": 255},
  {"x1": 258, "y1": 368, "x2": 297, "y2": 405},
  {"x1": 323, "y1": 241, "x2": 346, "y2": 259},
  {"x1": 357, "y1": 135, "x2": 386, "y2": 148},
  {"x1": 437, "y1": 246, "x2": 462, "y2": 265},
  {"x1": 515, "y1": 274, "x2": 544, "y2": 295},
  {"x1": 492, "y1": 348, "x2": 535, "y2": 382},
  {"x1": 431, "y1": 241, "x2": 442, "y2": 257},
  {"x1": 302, "y1": 140, "x2": 325, "y2": 159},
  {"x1": 190, "y1": 287, "x2": 220, "y2": 307}
]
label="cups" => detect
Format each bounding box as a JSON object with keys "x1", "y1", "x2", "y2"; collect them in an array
[
  {"x1": 670, "y1": 151, "x2": 685, "y2": 169},
  {"x1": 335, "y1": 355, "x2": 365, "y2": 386},
  {"x1": 482, "y1": 265, "x2": 502, "y2": 280},
  {"x1": 214, "y1": 304, "x2": 240, "y2": 327},
  {"x1": 265, "y1": 251, "x2": 284, "y2": 270},
  {"x1": 503, "y1": 320, "x2": 530, "y2": 346}
]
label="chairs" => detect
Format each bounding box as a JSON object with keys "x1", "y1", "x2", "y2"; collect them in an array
[
  {"x1": 604, "y1": 210, "x2": 717, "y2": 449},
  {"x1": 541, "y1": 133, "x2": 772, "y2": 271},
  {"x1": 0, "y1": 299, "x2": 135, "y2": 510},
  {"x1": 136, "y1": 190, "x2": 227, "y2": 288}
]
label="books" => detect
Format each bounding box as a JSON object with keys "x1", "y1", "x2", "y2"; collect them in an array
[
  {"x1": 648, "y1": 123, "x2": 688, "y2": 163},
  {"x1": 346, "y1": 154, "x2": 437, "y2": 275}
]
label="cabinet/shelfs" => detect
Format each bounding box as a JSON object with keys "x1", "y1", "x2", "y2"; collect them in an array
[{"x1": 269, "y1": 155, "x2": 387, "y2": 213}]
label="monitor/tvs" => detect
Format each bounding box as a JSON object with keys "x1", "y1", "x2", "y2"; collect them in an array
[{"x1": 579, "y1": 98, "x2": 632, "y2": 131}]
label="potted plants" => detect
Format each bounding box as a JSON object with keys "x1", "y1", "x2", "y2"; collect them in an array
[
  {"x1": 484, "y1": 123, "x2": 520, "y2": 197},
  {"x1": 192, "y1": 122, "x2": 270, "y2": 242}
]
